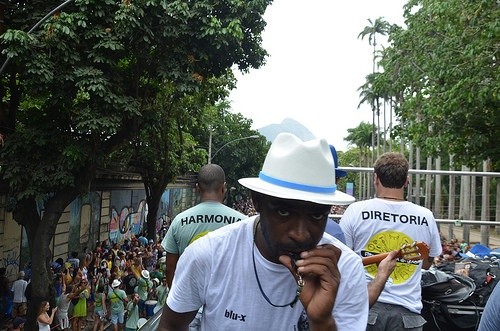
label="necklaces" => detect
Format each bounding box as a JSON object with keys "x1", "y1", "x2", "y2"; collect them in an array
[
  {"x1": 376, "y1": 195, "x2": 406, "y2": 201},
  {"x1": 251, "y1": 219, "x2": 304, "y2": 307}
]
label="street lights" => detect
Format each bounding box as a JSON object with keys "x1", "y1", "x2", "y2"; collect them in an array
[{"x1": 205, "y1": 127, "x2": 260, "y2": 164}]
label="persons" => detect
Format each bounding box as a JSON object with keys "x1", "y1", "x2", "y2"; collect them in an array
[
  {"x1": 233, "y1": 198, "x2": 260, "y2": 216},
  {"x1": 432, "y1": 234, "x2": 500, "y2": 277},
  {"x1": 328, "y1": 205, "x2": 347, "y2": 224},
  {"x1": 477, "y1": 280, "x2": 500, "y2": 331},
  {"x1": 324, "y1": 145, "x2": 348, "y2": 245},
  {"x1": 161, "y1": 164, "x2": 250, "y2": 331},
  {"x1": 0, "y1": 217, "x2": 172, "y2": 331},
  {"x1": 339, "y1": 151, "x2": 442, "y2": 331},
  {"x1": 157, "y1": 133, "x2": 369, "y2": 331}
]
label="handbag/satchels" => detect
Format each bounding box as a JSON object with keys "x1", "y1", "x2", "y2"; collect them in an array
[
  {"x1": 123, "y1": 299, "x2": 128, "y2": 309},
  {"x1": 71, "y1": 298, "x2": 79, "y2": 305},
  {"x1": 420, "y1": 271, "x2": 480, "y2": 331},
  {"x1": 468, "y1": 264, "x2": 500, "y2": 308}
]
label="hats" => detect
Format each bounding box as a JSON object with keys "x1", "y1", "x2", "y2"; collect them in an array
[
  {"x1": 111, "y1": 279, "x2": 121, "y2": 288},
  {"x1": 238, "y1": 130, "x2": 356, "y2": 206},
  {"x1": 18, "y1": 271, "x2": 25, "y2": 278},
  {"x1": 152, "y1": 278, "x2": 160, "y2": 286},
  {"x1": 141, "y1": 270, "x2": 150, "y2": 279}
]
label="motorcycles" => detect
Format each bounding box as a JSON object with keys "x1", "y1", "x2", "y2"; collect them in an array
[{"x1": 421, "y1": 252, "x2": 500, "y2": 331}]
